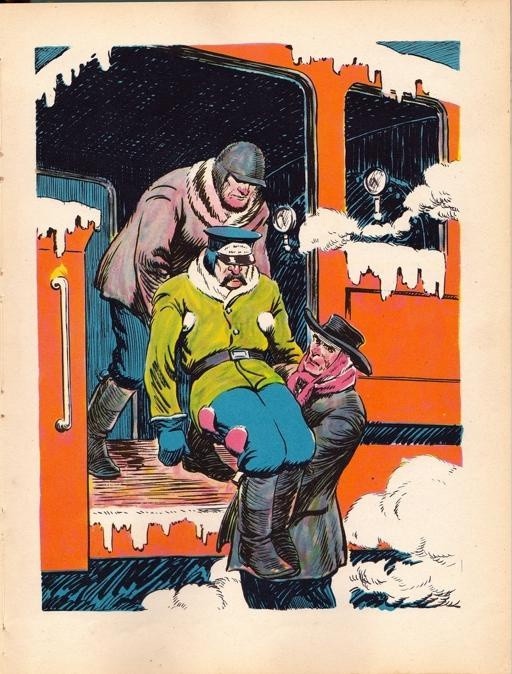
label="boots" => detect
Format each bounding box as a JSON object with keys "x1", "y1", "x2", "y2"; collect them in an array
[
  {"x1": 84, "y1": 369, "x2": 145, "y2": 480},
  {"x1": 184, "y1": 417, "x2": 235, "y2": 483},
  {"x1": 237, "y1": 461, "x2": 308, "y2": 580}
]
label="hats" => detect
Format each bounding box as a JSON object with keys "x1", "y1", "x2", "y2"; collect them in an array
[
  {"x1": 203, "y1": 225, "x2": 263, "y2": 266},
  {"x1": 213, "y1": 140, "x2": 267, "y2": 193},
  {"x1": 303, "y1": 306, "x2": 373, "y2": 377}
]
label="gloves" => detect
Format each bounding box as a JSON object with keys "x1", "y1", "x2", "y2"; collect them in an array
[
  {"x1": 148, "y1": 412, "x2": 190, "y2": 468},
  {"x1": 197, "y1": 405, "x2": 221, "y2": 436},
  {"x1": 181, "y1": 309, "x2": 196, "y2": 332},
  {"x1": 258, "y1": 311, "x2": 276, "y2": 335},
  {"x1": 224, "y1": 423, "x2": 248, "y2": 458}
]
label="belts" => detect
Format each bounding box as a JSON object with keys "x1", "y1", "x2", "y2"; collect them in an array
[{"x1": 188, "y1": 348, "x2": 266, "y2": 380}]
